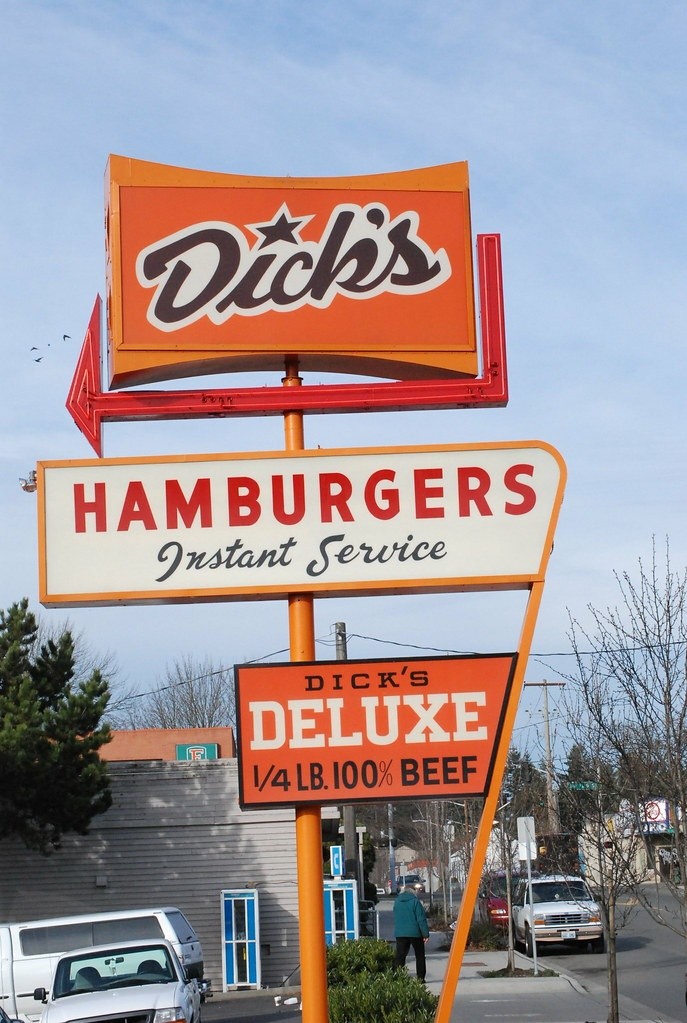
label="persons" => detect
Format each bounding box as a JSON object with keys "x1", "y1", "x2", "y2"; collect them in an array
[{"x1": 393, "y1": 886, "x2": 429, "y2": 983}]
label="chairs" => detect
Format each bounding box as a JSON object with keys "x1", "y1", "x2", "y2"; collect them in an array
[
  {"x1": 136, "y1": 959, "x2": 164, "y2": 983},
  {"x1": 70, "y1": 966, "x2": 104, "y2": 994}
]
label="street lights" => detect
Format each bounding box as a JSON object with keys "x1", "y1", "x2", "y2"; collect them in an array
[
  {"x1": 430, "y1": 800, "x2": 472, "y2": 870},
  {"x1": 412, "y1": 818, "x2": 443, "y2": 893}
]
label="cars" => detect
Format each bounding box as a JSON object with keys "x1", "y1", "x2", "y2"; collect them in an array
[
  {"x1": 31, "y1": 937, "x2": 202, "y2": 1023},
  {"x1": 376, "y1": 887, "x2": 385, "y2": 895}
]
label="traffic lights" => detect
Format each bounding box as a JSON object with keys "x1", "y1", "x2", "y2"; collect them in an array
[{"x1": 502, "y1": 793, "x2": 514, "y2": 811}]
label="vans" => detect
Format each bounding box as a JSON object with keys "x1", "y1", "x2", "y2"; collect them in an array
[{"x1": 0, "y1": 906, "x2": 213, "y2": 1023}]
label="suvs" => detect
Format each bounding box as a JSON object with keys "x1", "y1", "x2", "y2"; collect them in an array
[
  {"x1": 395, "y1": 874, "x2": 426, "y2": 895},
  {"x1": 511, "y1": 874, "x2": 606, "y2": 958},
  {"x1": 476, "y1": 866, "x2": 543, "y2": 937}
]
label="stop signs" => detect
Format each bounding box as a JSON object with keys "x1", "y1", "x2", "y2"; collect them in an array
[{"x1": 388, "y1": 880, "x2": 392, "y2": 886}]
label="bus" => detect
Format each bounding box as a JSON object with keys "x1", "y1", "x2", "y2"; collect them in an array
[{"x1": 517, "y1": 832, "x2": 589, "y2": 881}]
